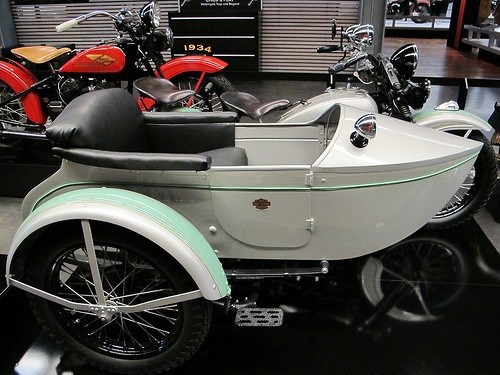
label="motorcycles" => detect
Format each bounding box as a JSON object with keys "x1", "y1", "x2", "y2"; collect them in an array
[
  {"x1": 1, "y1": 16, "x2": 498, "y2": 374},
  {"x1": 0, "y1": 2, "x2": 242, "y2": 150}
]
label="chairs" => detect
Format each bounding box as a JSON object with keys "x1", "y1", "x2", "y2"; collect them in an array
[{"x1": 45, "y1": 87, "x2": 249, "y2": 167}]
